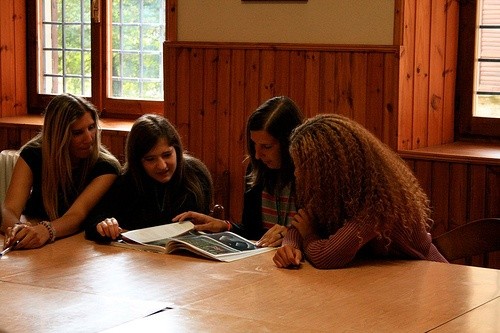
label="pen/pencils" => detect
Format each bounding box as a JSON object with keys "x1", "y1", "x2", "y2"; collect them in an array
[{"x1": 0, "y1": 241, "x2": 20, "y2": 256}]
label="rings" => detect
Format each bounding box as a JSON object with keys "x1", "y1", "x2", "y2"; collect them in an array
[{"x1": 279, "y1": 233, "x2": 283, "y2": 238}]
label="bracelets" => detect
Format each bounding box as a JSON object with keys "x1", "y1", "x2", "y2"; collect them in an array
[
  {"x1": 33, "y1": 221, "x2": 56, "y2": 244},
  {"x1": 226, "y1": 220, "x2": 230, "y2": 231}
]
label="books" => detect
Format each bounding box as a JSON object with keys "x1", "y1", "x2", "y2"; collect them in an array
[{"x1": 109, "y1": 220, "x2": 284, "y2": 263}]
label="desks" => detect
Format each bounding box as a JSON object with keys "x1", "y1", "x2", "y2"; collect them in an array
[{"x1": 0, "y1": 214, "x2": 500, "y2": 333}]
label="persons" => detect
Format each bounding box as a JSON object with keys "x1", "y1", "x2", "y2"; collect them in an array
[
  {"x1": 172, "y1": 95, "x2": 303, "y2": 247},
  {"x1": 0, "y1": 93, "x2": 122, "y2": 249},
  {"x1": 84, "y1": 114, "x2": 212, "y2": 245},
  {"x1": 273, "y1": 113, "x2": 449, "y2": 269}
]
